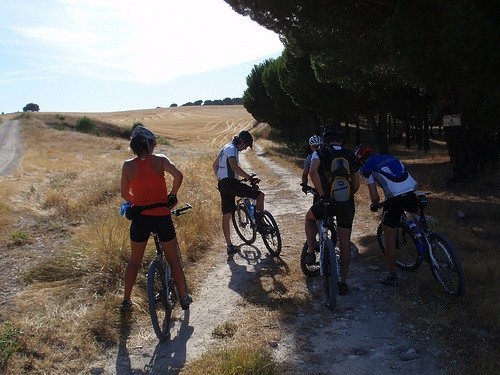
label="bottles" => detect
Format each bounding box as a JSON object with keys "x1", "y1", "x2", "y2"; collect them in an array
[
  {"x1": 248, "y1": 203, "x2": 255, "y2": 217},
  {"x1": 408, "y1": 221, "x2": 421, "y2": 239}
]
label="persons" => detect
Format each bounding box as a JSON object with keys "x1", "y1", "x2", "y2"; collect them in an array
[
  {"x1": 354, "y1": 143, "x2": 444, "y2": 286},
  {"x1": 212, "y1": 130, "x2": 273, "y2": 253},
  {"x1": 301, "y1": 124, "x2": 361, "y2": 295},
  {"x1": 119, "y1": 126, "x2": 193, "y2": 311}
]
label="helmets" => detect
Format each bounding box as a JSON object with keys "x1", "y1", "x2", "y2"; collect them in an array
[
  {"x1": 130, "y1": 125, "x2": 155, "y2": 141},
  {"x1": 239, "y1": 131, "x2": 253, "y2": 149},
  {"x1": 354, "y1": 144, "x2": 376, "y2": 160},
  {"x1": 308, "y1": 135, "x2": 325, "y2": 145},
  {"x1": 322, "y1": 127, "x2": 344, "y2": 140}
]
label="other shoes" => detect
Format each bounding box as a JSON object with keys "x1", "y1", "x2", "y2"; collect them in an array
[
  {"x1": 380, "y1": 275, "x2": 399, "y2": 286},
  {"x1": 179, "y1": 293, "x2": 193, "y2": 310},
  {"x1": 338, "y1": 280, "x2": 348, "y2": 295},
  {"x1": 119, "y1": 299, "x2": 132, "y2": 310},
  {"x1": 227, "y1": 244, "x2": 240, "y2": 253},
  {"x1": 304, "y1": 252, "x2": 316, "y2": 264},
  {"x1": 256, "y1": 213, "x2": 267, "y2": 224}
]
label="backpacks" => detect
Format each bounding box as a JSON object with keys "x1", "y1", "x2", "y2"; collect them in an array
[{"x1": 317, "y1": 145, "x2": 354, "y2": 205}]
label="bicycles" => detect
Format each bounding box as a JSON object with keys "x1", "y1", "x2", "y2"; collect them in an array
[
  {"x1": 378, "y1": 200, "x2": 465, "y2": 296},
  {"x1": 232, "y1": 171, "x2": 282, "y2": 257},
  {"x1": 300, "y1": 182, "x2": 338, "y2": 310},
  {"x1": 146, "y1": 202, "x2": 193, "y2": 342}
]
